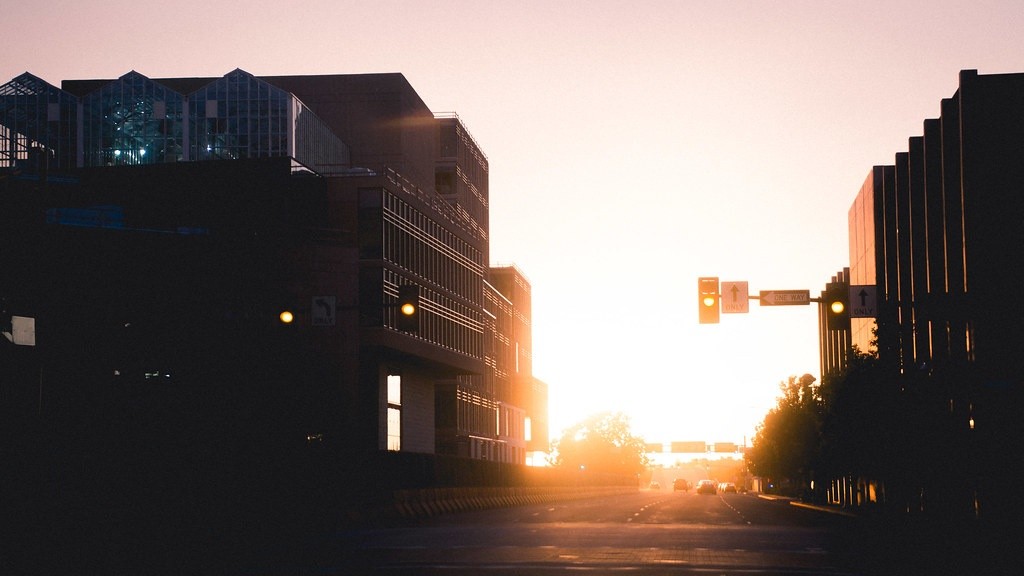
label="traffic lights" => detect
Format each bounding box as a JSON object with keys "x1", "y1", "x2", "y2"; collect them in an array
[
  {"x1": 278, "y1": 298, "x2": 298, "y2": 331},
  {"x1": 697, "y1": 276, "x2": 721, "y2": 324},
  {"x1": 826, "y1": 282, "x2": 851, "y2": 330},
  {"x1": 398, "y1": 284, "x2": 420, "y2": 332}
]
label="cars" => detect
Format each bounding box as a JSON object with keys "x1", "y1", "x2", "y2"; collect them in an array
[{"x1": 673, "y1": 478, "x2": 737, "y2": 495}]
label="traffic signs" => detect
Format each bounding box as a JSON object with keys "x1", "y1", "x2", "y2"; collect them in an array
[
  {"x1": 849, "y1": 285, "x2": 878, "y2": 318},
  {"x1": 720, "y1": 281, "x2": 750, "y2": 313},
  {"x1": 312, "y1": 295, "x2": 336, "y2": 327},
  {"x1": 759, "y1": 289, "x2": 811, "y2": 306}
]
